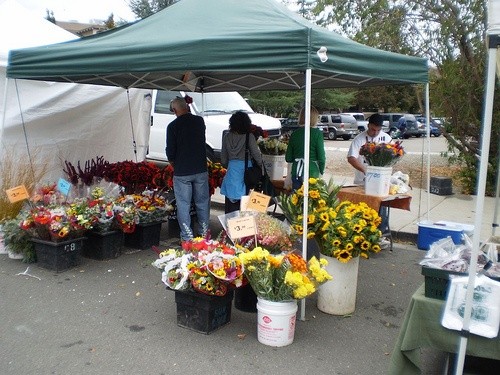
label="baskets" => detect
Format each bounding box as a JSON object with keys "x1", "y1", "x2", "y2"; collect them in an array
[{"x1": 419, "y1": 265, "x2": 468, "y2": 300}]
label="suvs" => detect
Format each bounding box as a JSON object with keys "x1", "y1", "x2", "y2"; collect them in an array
[
  {"x1": 367, "y1": 112, "x2": 419, "y2": 139},
  {"x1": 319, "y1": 113, "x2": 360, "y2": 139}
]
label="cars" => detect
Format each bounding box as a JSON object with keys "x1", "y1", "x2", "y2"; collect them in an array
[
  {"x1": 414, "y1": 114, "x2": 453, "y2": 137},
  {"x1": 278, "y1": 116, "x2": 329, "y2": 137}
]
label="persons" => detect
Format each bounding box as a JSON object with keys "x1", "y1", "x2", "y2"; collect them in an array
[
  {"x1": 346, "y1": 112, "x2": 395, "y2": 184},
  {"x1": 286, "y1": 105, "x2": 325, "y2": 192},
  {"x1": 221, "y1": 111, "x2": 262, "y2": 214},
  {"x1": 166, "y1": 97, "x2": 211, "y2": 245}
]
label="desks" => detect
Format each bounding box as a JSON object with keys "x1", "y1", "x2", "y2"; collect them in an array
[
  {"x1": 269, "y1": 177, "x2": 413, "y2": 254},
  {"x1": 392, "y1": 286, "x2": 500, "y2": 375}
]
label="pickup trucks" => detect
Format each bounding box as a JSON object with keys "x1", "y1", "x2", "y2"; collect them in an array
[{"x1": 340, "y1": 113, "x2": 390, "y2": 134}]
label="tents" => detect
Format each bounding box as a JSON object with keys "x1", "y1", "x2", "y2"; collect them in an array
[
  {"x1": 0, "y1": 0, "x2": 430, "y2": 322},
  {"x1": 0, "y1": 0, "x2": 153, "y2": 211}
]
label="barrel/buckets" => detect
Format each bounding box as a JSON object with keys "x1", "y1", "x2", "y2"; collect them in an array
[
  {"x1": 255, "y1": 297, "x2": 299, "y2": 347},
  {"x1": 262, "y1": 154, "x2": 285, "y2": 180},
  {"x1": 366, "y1": 165, "x2": 392, "y2": 197}
]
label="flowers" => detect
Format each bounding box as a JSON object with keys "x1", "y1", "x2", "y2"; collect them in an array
[
  {"x1": 250, "y1": 124, "x2": 288, "y2": 156},
  {"x1": 150, "y1": 178, "x2": 383, "y2": 301},
  {"x1": 359, "y1": 135, "x2": 407, "y2": 166},
  {"x1": 0, "y1": 155, "x2": 174, "y2": 263},
  {"x1": 207, "y1": 161, "x2": 227, "y2": 194}
]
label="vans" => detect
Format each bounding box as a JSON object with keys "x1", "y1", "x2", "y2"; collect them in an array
[{"x1": 144, "y1": 92, "x2": 282, "y2": 164}]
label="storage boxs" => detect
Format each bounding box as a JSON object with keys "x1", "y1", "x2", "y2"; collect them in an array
[
  {"x1": 82, "y1": 229, "x2": 124, "y2": 259},
  {"x1": 420, "y1": 252, "x2": 500, "y2": 300},
  {"x1": 431, "y1": 176, "x2": 455, "y2": 196},
  {"x1": 28, "y1": 235, "x2": 90, "y2": 273},
  {"x1": 167, "y1": 216, "x2": 193, "y2": 236},
  {"x1": 124, "y1": 221, "x2": 162, "y2": 249},
  {"x1": 418, "y1": 219, "x2": 474, "y2": 250},
  {"x1": 167, "y1": 287, "x2": 233, "y2": 334}
]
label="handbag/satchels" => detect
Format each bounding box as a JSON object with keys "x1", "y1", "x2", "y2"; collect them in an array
[
  {"x1": 261, "y1": 162, "x2": 274, "y2": 197},
  {"x1": 244, "y1": 167, "x2": 259, "y2": 189}
]
